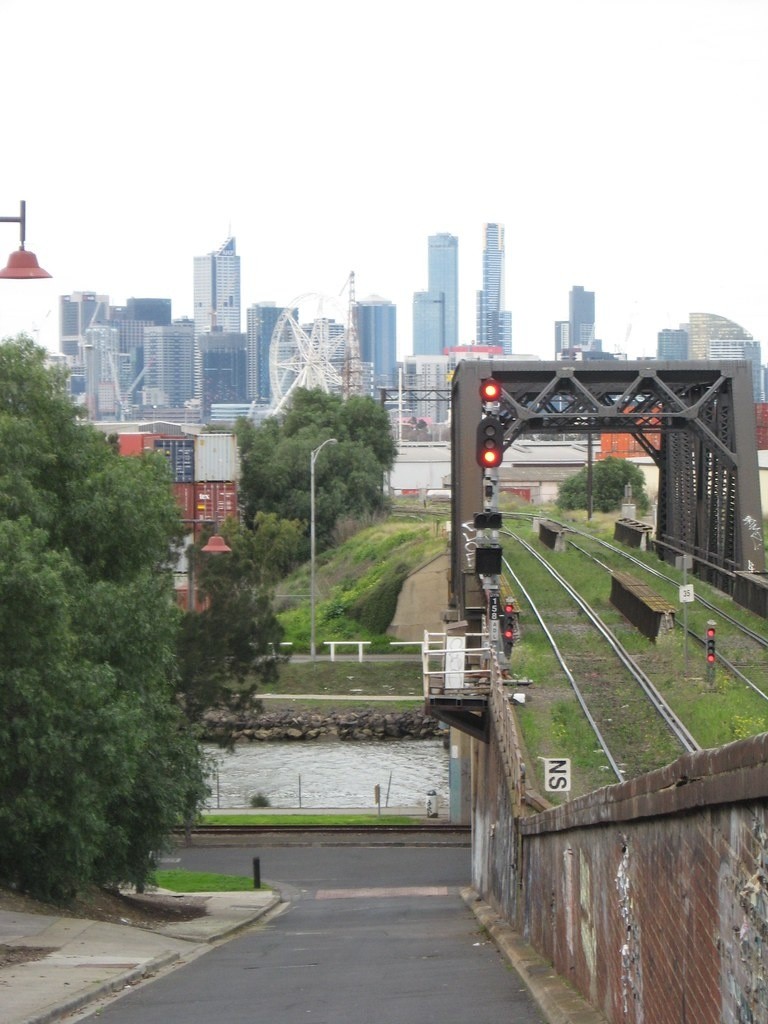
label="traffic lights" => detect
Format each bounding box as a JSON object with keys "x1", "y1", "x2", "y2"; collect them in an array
[
  {"x1": 476, "y1": 418, "x2": 503, "y2": 467},
  {"x1": 478, "y1": 378, "x2": 500, "y2": 400},
  {"x1": 706, "y1": 627, "x2": 716, "y2": 660},
  {"x1": 503, "y1": 604, "x2": 515, "y2": 641}
]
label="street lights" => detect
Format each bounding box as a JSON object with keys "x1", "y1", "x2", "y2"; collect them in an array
[
  {"x1": 311, "y1": 439, "x2": 340, "y2": 654},
  {"x1": 188, "y1": 518, "x2": 233, "y2": 609}
]
